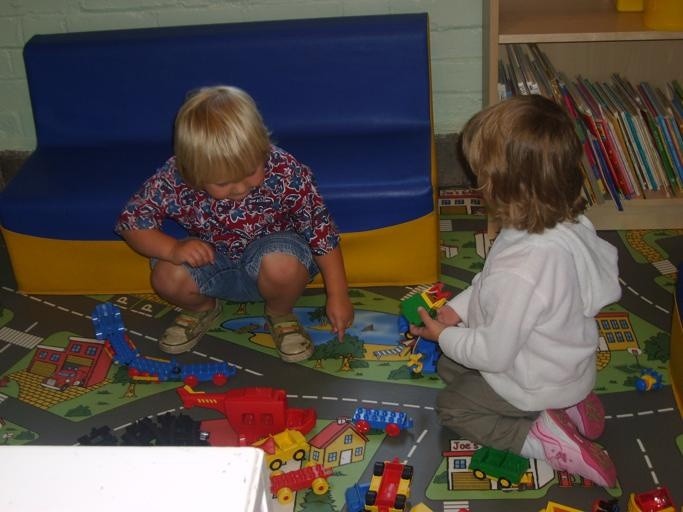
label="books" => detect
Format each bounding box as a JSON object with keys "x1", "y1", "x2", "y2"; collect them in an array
[{"x1": 500, "y1": 43, "x2": 683, "y2": 212}]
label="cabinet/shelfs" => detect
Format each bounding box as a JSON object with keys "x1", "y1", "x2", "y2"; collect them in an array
[{"x1": 481, "y1": 2, "x2": 683, "y2": 233}]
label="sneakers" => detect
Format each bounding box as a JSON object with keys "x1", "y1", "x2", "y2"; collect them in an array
[
  {"x1": 158, "y1": 298, "x2": 223, "y2": 355},
  {"x1": 262, "y1": 305, "x2": 314, "y2": 363},
  {"x1": 529, "y1": 409, "x2": 616, "y2": 488},
  {"x1": 564, "y1": 392, "x2": 605, "y2": 441}
]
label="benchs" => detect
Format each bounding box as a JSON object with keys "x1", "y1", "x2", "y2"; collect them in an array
[{"x1": 1, "y1": 11, "x2": 441, "y2": 296}]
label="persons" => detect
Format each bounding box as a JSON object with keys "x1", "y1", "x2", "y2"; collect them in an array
[
  {"x1": 114, "y1": 84, "x2": 354, "y2": 362},
  {"x1": 409, "y1": 91, "x2": 618, "y2": 490}
]
